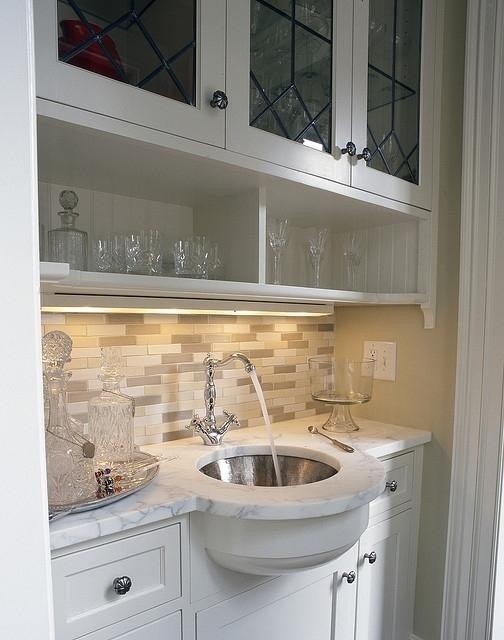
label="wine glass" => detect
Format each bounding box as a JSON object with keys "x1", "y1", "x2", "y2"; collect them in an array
[
  {"x1": 339, "y1": 230, "x2": 368, "y2": 292},
  {"x1": 301, "y1": 227, "x2": 330, "y2": 288},
  {"x1": 267, "y1": 216, "x2": 292, "y2": 285}
]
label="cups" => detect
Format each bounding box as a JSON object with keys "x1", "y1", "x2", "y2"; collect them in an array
[
  {"x1": 250, "y1": 82, "x2": 327, "y2": 148},
  {"x1": 124, "y1": 230, "x2": 163, "y2": 276},
  {"x1": 94, "y1": 232, "x2": 125, "y2": 273},
  {"x1": 171, "y1": 234, "x2": 219, "y2": 280}
]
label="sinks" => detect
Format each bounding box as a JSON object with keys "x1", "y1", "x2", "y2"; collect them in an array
[{"x1": 196, "y1": 443, "x2": 342, "y2": 487}]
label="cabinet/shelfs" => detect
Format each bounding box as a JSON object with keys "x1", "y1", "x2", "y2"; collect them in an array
[
  {"x1": 358, "y1": 443, "x2": 427, "y2": 640},
  {"x1": 195, "y1": 544, "x2": 359, "y2": 640},
  {"x1": 37, "y1": 96, "x2": 435, "y2": 302},
  {"x1": 49, "y1": 514, "x2": 194, "y2": 640},
  {"x1": 32, "y1": 0, "x2": 226, "y2": 147},
  {"x1": 229, "y1": 1, "x2": 444, "y2": 213}
]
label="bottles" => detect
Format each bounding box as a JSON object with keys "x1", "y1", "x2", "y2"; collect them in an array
[
  {"x1": 41, "y1": 330, "x2": 98, "y2": 506},
  {"x1": 47, "y1": 190, "x2": 87, "y2": 271},
  {"x1": 87, "y1": 349, "x2": 134, "y2": 462}
]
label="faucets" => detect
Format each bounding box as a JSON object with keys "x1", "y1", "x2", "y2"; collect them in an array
[{"x1": 184, "y1": 351, "x2": 257, "y2": 446}]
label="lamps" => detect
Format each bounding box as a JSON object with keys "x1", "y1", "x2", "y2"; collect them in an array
[{"x1": 41, "y1": 297, "x2": 335, "y2": 317}]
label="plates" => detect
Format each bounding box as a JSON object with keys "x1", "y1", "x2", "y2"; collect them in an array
[{"x1": 50, "y1": 452, "x2": 159, "y2": 517}]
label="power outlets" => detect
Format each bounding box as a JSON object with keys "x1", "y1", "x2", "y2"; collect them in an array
[{"x1": 360, "y1": 341, "x2": 377, "y2": 379}]
